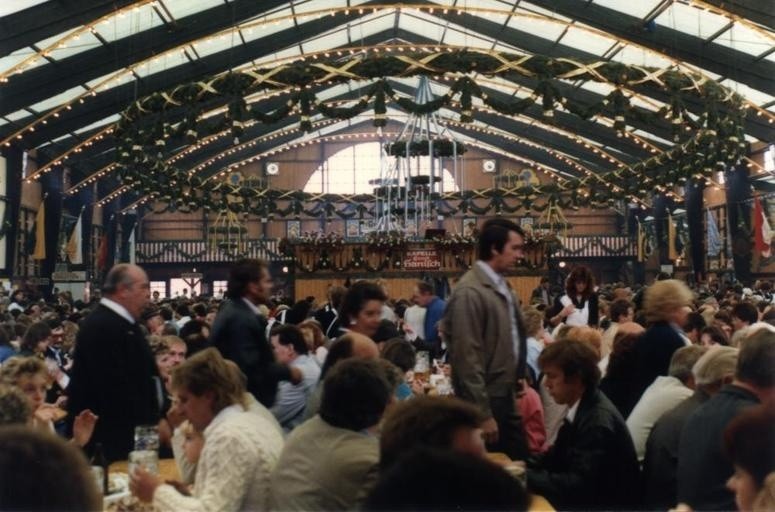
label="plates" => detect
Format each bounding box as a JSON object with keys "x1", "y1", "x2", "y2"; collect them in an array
[
  {"x1": 105, "y1": 471, "x2": 128, "y2": 493},
  {"x1": 103, "y1": 493, "x2": 168, "y2": 511}
]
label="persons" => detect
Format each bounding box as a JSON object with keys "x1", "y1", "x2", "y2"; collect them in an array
[
  {"x1": 1, "y1": 260, "x2": 775, "y2": 512},
  {"x1": 442, "y1": 214, "x2": 528, "y2": 452}
]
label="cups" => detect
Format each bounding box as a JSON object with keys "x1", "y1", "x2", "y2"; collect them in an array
[
  {"x1": 506, "y1": 466, "x2": 527, "y2": 487},
  {"x1": 135, "y1": 425, "x2": 161, "y2": 462},
  {"x1": 127, "y1": 450, "x2": 158, "y2": 477},
  {"x1": 413, "y1": 350, "x2": 430, "y2": 384}
]
